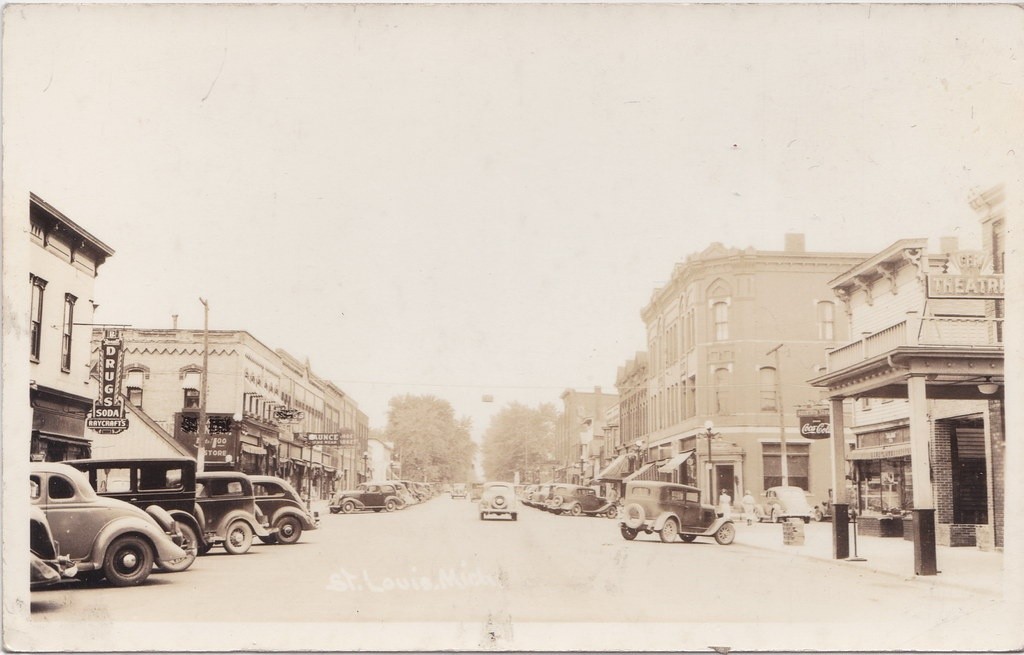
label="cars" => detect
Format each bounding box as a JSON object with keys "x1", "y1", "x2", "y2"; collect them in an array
[
  {"x1": 30, "y1": 463, "x2": 186, "y2": 586},
  {"x1": 242, "y1": 477, "x2": 316, "y2": 544},
  {"x1": 450, "y1": 483, "x2": 468, "y2": 499},
  {"x1": 814, "y1": 488, "x2": 858, "y2": 523},
  {"x1": 329, "y1": 480, "x2": 449, "y2": 513},
  {"x1": 171, "y1": 472, "x2": 270, "y2": 555},
  {"x1": 620, "y1": 480, "x2": 735, "y2": 545},
  {"x1": 478, "y1": 481, "x2": 519, "y2": 521},
  {"x1": 469, "y1": 484, "x2": 484, "y2": 501},
  {"x1": 515, "y1": 484, "x2": 618, "y2": 518},
  {"x1": 58, "y1": 459, "x2": 203, "y2": 571},
  {"x1": 753, "y1": 485, "x2": 812, "y2": 524}
]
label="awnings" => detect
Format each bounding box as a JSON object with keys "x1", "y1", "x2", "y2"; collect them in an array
[
  {"x1": 597, "y1": 454, "x2": 633, "y2": 480},
  {"x1": 657, "y1": 449, "x2": 695, "y2": 472}
]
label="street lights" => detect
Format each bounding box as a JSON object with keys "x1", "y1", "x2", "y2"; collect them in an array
[
  {"x1": 228, "y1": 412, "x2": 246, "y2": 473},
  {"x1": 575, "y1": 455, "x2": 590, "y2": 486},
  {"x1": 696, "y1": 420, "x2": 723, "y2": 506},
  {"x1": 632, "y1": 441, "x2": 643, "y2": 469},
  {"x1": 304, "y1": 435, "x2": 315, "y2": 513},
  {"x1": 363, "y1": 451, "x2": 368, "y2": 483}
]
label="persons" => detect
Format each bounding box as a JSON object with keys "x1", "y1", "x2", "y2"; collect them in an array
[
  {"x1": 740, "y1": 490, "x2": 758, "y2": 527},
  {"x1": 717, "y1": 489, "x2": 731, "y2": 518}
]
